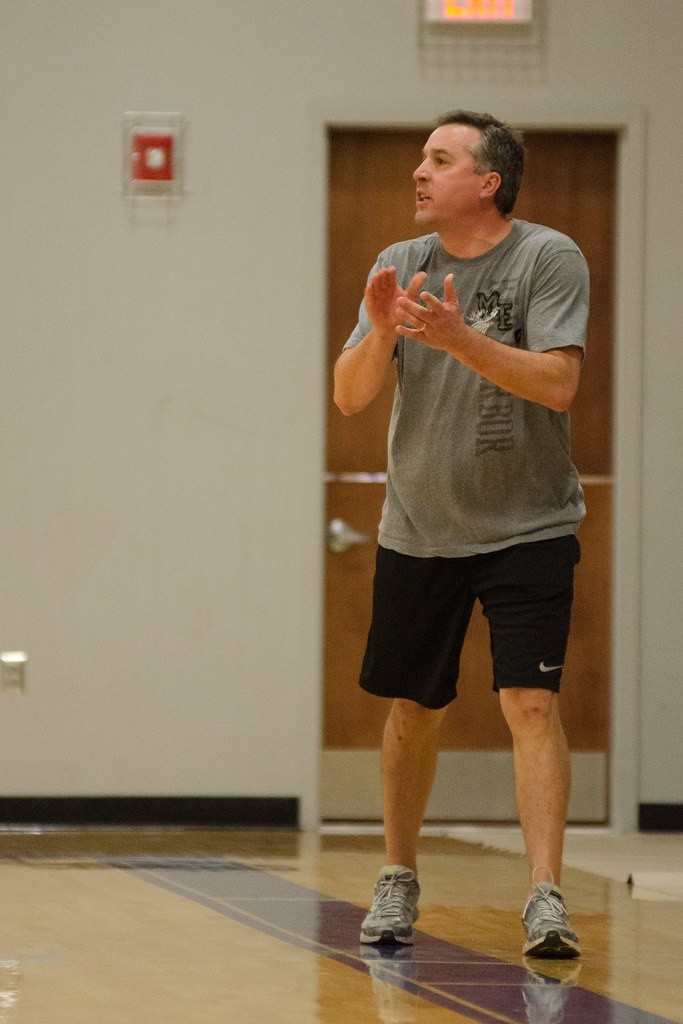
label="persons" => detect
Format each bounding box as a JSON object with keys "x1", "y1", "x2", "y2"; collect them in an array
[{"x1": 334, "y1": 109, "x2": 591, "y2": 958}]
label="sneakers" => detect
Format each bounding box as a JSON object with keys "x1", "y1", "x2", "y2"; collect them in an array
[
  {"x1": 520, "y1": 957, "x2": 581, "y2": 1024},
  {"x1": 522, "y1": 865, "x2": 581, "y2": 960},
  {"x1": 360, "y1": 943, "x2": 415, "y2": 1022},
  {"x1": 360, "y1": 867, "x2": 420, "y2": 945}
]
